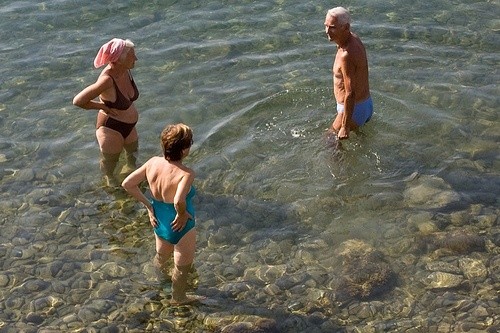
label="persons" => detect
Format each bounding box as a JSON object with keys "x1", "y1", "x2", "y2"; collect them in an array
[
  {"x1": 122, "y1": 123, "x2": 201, "y2": 305},
  {"x1": 73, "y1": 39, "x2": 139, "y2": 187},
  {"x1": 324, "y1": 7, "x2": 374, "y2": 140}
]
